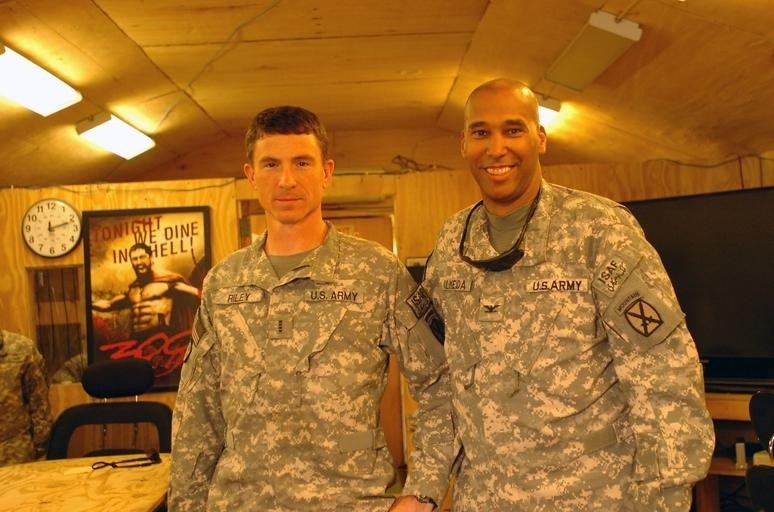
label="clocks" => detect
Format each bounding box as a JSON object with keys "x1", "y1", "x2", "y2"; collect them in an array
[{"x1": 22, "y1": 197, "x2": 83, "y2": 258}]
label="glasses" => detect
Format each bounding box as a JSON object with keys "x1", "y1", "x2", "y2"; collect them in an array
[
  {"x1": 92, "y1": 448, "x2": 161, "y2": 469},
  {"x1": 459, "y1": 187, "x2": 540, "y2": 271}
]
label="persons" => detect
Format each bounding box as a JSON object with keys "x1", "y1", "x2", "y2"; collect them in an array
[
  {"x1": 166, "y1": 105, "x2": 455, "y2": 512},
  {"x1": 92, "y1": 243, "x2": 201, "y2": 343},
  {"x1": 422, "y1": 77, "x2": 717, "y2": 512}
]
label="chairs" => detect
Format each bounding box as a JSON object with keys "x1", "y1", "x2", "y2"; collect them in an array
[
  {"x1": 49, "y1": 357, "x2": 173, "y2": 459},
  {"x1": 742, "y1": 391, "x2": 774, "y2": 511}
]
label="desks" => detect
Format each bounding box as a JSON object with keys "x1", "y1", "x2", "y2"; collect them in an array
[{"x1": 0, "y1": 453, "x2": 172, "y2": 512}]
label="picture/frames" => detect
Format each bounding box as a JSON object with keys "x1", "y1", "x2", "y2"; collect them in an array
[{"x1": 83, "y1": 207, "x2": 212, "y2": 393}]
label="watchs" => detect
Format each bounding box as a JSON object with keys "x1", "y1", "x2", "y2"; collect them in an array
[{"x1": 415, "y1": 495, "x2": 435, "y2": 503}]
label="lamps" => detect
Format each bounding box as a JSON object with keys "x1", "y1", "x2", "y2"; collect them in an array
[{"x1": 0, "y1": 43, "x2": 156, "y2": 163}]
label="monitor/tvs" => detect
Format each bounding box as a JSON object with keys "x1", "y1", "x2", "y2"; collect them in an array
[{"x1": 618, "y1": 185, "x2": 774, "y2": 393}]
